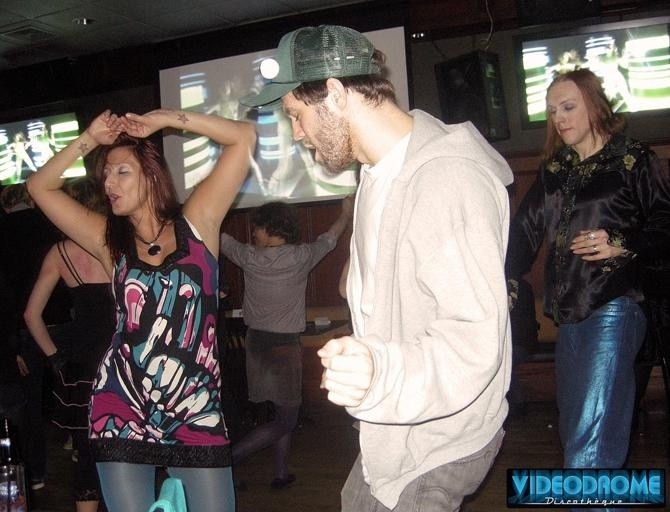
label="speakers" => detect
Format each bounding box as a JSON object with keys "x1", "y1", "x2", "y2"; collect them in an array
[{"x1": 434, "y1": 49, "x2": 510, "y2": 142}]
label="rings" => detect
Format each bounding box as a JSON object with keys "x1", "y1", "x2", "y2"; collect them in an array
[
  {"x1": 592, "y1": 245, "x2": 597, "y2": 253},
  {"x1": 584, "y1": 232, "x2": 594, "y2": 240}
]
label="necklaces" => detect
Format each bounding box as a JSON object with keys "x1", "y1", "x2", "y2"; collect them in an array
[{"x1": 134, "y1": 218, "x2": 169, "y2": 259}]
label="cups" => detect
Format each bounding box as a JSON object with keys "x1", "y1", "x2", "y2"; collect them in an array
[{"x1": 0, "y1": 465, "x2": 27, "y2": 511}]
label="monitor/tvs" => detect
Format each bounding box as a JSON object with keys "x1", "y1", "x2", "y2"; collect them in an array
[
  {"x1": 512, "y1": 15, "x2": 670, "y2": 130},
  {"x1": 0, "y1": 105, "x2": 93, "y2": 190}
]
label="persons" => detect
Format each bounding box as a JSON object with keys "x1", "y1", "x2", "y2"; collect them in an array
[
  {"x1": 202, "y1": 81, "x2": 271, "y2": 198},
  {"x1": 7, "y1": 132, "x2": 39, "y2": 183},
  {"x1": 501, "y1": 64, "x2": 669, "y2": 471},
  {"x1": 21, "y1": 182, "x2": 119, "y2": 510},
  {"x1": 588, "y1": 43, "x2": 635, "y2": 112},
  {"x1": 29, "y1": 127, "x2": 56, "y2": 163},
  {"x1": 0, "y1": 183, "x2": 68, "y2": 490},
  {"x1": 545, "y1": 49, "x2": 591, "y2": 74},
  {"x1": 220, "y1": 192, "x2": 354, "y2": 491},
  {"x1": 242, "y1": 23, "x2": 524, "y2": 511},
  {"x1": 266, "y1": 104, "x2": 317, "y2": 193},
  {"x1": 23, "y1": 109, "x2": 258, "y2": 511}
]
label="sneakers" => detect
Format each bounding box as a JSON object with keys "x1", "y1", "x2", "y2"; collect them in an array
[{"x1": 233, "y1": 474, "x2": 295, "y2": 489}]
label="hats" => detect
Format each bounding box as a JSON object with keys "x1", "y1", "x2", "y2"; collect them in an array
[{"x1": 238, "y1": 25, "x2": 384, "y2": 108}]
